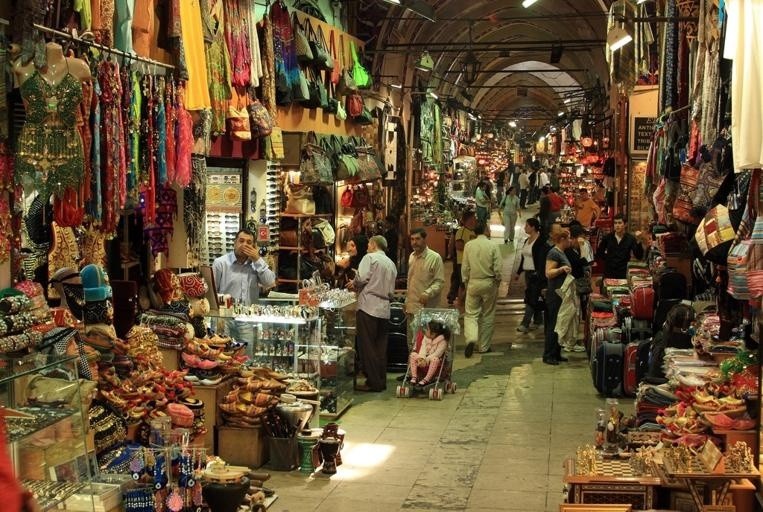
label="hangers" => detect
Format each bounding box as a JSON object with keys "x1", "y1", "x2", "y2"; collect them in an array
[
  {"x1": 80, "y1": 38, "x2": 90, "y2": 64},
  {"x1": 148, "y1": 57, "x2": 152, "y2": 80},
  {"x1": 171, "y1": 65, "x2": 174, "y2": 82},
  {"x1": 142, "y1": 56, "x2": 146, "y2": 80},
  {"x1": 154, "y1": 59, "x2": 157, "y2": 77},
  {"x1": 277, "y1": 0, "x2": 287, "y2": 8},
  {"x1": 121, "y1": 51, "x2": 126, "y2": 66},
  {"x1": 89, "y1": 40, "x2": 99, "y2": 63},
  {"x1": 158, "y1": 62, "x2": 162, "y2": 78},
  {"x1": 107, "y1": 47, "x2": 113, "y2": 63},
  {"x1": 97, "y1": 44, "x2": 106, "y2": 62},
  {"x1": 0, "y1": 352, "x2": 95, "y2": 512},
  {"x1": 166, "y1": 64, "x2": 168, "y2": 84},
  {"x1": 127, "y1": 53, "x2": 134, "y2": 72},
  {"x1": 136, "y1": 54, "x2": 140, "y2": 72},
  {"x1": 111, "y1": 48, "x2": 119, "y2": 64}
]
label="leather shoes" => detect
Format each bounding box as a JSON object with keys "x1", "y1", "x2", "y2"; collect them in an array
[{"x1": 356, "y1": 383, "x2": 381, "y2": 392}]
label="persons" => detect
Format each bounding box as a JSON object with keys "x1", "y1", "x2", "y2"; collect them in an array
[
  {"x1": 473, "y1": 182, "x2": 491, "y2": 237},
  {"x1": 533, "y1": 185, "x2": 553, "y2": 246},
  {"x1": 339, "y1": 235, "x2": 368, "y2": 335},
  {"x1": 460, "y1": 221, "x2": 503, "y2": 357},
  {"x1": 397, "y1": 227, "x2": 445, "y2": 380},
  {"x1": 549, "y1": 186, "x2": 564, "y2": 222},
  {"x1": 345, "y1": 234, "x2": 397, "y2": 392},
  {"x1": 595, "y1": 212, "x2": 647, "y2": 294},
  {"x1": 514, "y1": 154, "x2": 557, "y2": 203},
  {"x1": 455, "y1": 212, "x2": 478, "y2": 313},
  {"x1": 497, "y1": 186, "x2": 522, "y2": 244},
  {"x1": 543, "y1": 224, "x2": 574, "y2": 366},
  {"x1": 409, "y1": 321, "x2": 450, "y2": 385},
  {"x1": 566, "y1": 225, "x2": 592, "y2": 352},
  {"x1": 212, "y1": 228, "x2": 276, "y2": 360},
  {"x1": 516, "y1": 218, "x2": 548, "y2": 333},
  {"x1": 574, "y1": 188, "x2": 599, "y2": 237},
  {"x1": 447, "y1": 221, "x2": 465, "y2": 305},
  {"x1": 579, "y1": 230, "x2": 592, "y2": 321}
]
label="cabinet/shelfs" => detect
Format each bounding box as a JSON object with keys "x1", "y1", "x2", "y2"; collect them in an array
[
  {"x1": 335, "y1": 180, "x2": 388, "y2": 277},
  {"x1": 279, "y1": 183, "x2": 334, "y2": 294},
  {"x1": 411, "y1": 219, "x2": 449, "y2": 260},
  {"x1": 218, "y1": 425, "x2": 269, "y2": 469},
  {"x1": 158, "y1": 345, "x2": 180, "y2": 372},
  {"x1": 257, "y1": 297, "x2": 356, "y2": 419},
  {"x1": 559, "y1": 141, "x2": 615, "y2": 215},
  {"x1": 234, "y1": 316, "x2": 320, "y2": 429},
  {"x1": 191, "y1": 377, "x2": 235, "y2": 455},
  {"x1": 712, "y1": 395, "x2": 759, "y2": 512},
  {"x1": 203, "y1": 310, "x2": 235, "y2": 339},
  {"x1": 563, "y1": 475, "x2": 661, "y2": 511}
]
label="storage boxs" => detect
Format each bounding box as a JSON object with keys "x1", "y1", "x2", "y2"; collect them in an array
[
  {"x1": 92, "y1": 474, "x2": 131, "y2": 490},
  {"x1": 65, "y1": 482, "x2": 123, "y2": 511}
]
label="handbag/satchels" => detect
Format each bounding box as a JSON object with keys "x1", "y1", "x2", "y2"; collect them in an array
[
  {"x1": 575, "y1": 278, "x2": 592, "y2": 295},
  {"x1": 292, "y1": 70, "x2": 309, "y2": 102},
  {"x1": 330, "y1": 135, "x2": 359, "y2": 180},
  {"x1": 350, "y1": 136, "x2": 381, "y2": 183},
  {"x1": 316, "y1": 70, "x2": 328, "y2": 108},
  {"x1": 336, "y1": 90, "x2": 346, "y2": 120},
  {"x1": 227, "y1": 86, "x2": 251, "y2": 140},
  {"x1": 690, "y1": 170, "x2": 749, "y2": 264},
  {"x1": 348, "y1": 92, "x2": 362, "y2": 117},
  {"x1": 355, "y1": 96, "x2": 372, "y2": 125},
  {"x1": 312, "y1": 220, "x2": 335, "y2": 246},
  {"x1": 301, "y1": 66, "x2": 321, "y2": 108},
  {"x1": 361, "y1": 137, "x2": 388, "y2": 176},
  {"x1": 245, "y1": 88, "x2": 271, "y2": 138},
  {"x1": 342, "y1": 184, "x2": 368, "y2": 208},
  {"x1": 300, "y1": 132, "x2": 334, "y2": 186},
  {"x1": 329, "y1": 30, "x2": 340, "y2": 84},
  {"x1": 285, "y1": 184, "x2": 316, "y2": 214},
  {"x1": 502, "y1": 197, "x2": 506, "y2": 206},
  {"x1": 325, "y1": 81, "x2": 337, "y2": 114},
  {"x1": 349, "y1": 41, "x2": 370, "y2": 88},
  {"x1": 339, "y1": 136, "x2": 368, "y2": 185},
  {"x1": 293, "y1": 12, "x2": 313, "y2": 61},
  {"x1": 304, "y1": 18, "x2": 326, "y2": 65},
  {"x1": 316, "y1": 26, "x2": 333, "y2": 70},
  {"x1": 341, "y1": 35, "x2": 357, "y2": 95},
  {"x1": 727, "y1": 168, "x2": 763, "y2": 300}
]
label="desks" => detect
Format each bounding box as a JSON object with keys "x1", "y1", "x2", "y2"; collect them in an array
[{"x1": 662, "y1": 455, "x2": 761, "y2": 512}]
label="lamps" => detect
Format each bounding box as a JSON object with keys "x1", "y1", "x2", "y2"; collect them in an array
[
  {"x1": 550, "y1": 42, "x2": 565, "y2": 64},
  {"x1": 522, "y1": 0, "x2": 537, "y2": 8},
  {"x1": 414, "y1": 47, "x2": 434, "y2": 73},
  {"x1": 557, "y1": 111, "x2": 577, "y2": 116},
  {"x1": 607, "y1": 17, "x2": 700, "y2": 50},
  {"x1": 563, "y1": 93, "x2": 584, "y2": 103},
  {"x1": 459, "y1": 21, "x2": 483, "y2": 90}
]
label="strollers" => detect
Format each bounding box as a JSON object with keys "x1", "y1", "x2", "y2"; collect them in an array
[{"x1": 396, "y1": 308, "x2": 458, "y2": 401}]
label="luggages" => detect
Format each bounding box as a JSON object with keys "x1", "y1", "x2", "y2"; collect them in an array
[
  {"x1": 591, "y1": 328, "x2": 620, "y2": 347},
  {"x1": 624, "y1": 342, "x2": 640, "y2": 397},
  {"x1": 592, "y1": 341, "x2": 622, "y2": 396}
]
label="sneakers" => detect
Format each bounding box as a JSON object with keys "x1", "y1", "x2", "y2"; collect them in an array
[
  {"x1": 544, "y1": 357, "x2": 558, "y2": 364},
  {"x1": 557, "y1": 355, "x2": 567, "y2": 361},
  {"x1": 530, "y1": 323, "x2": 545, "y2": 330},
  {"x1": 565, "y1": 344, "x2": 585, "y2": 352},
  {"x1": 517, "y1": 325, "x2": 529, "y2": 333}
]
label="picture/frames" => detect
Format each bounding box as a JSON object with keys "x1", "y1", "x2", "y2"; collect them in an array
[
  {"x1": 631, "y1": 114, "x2": 657, "y2": 155},
  {"x1": 671, "y1": 492, "x2": 703, "y2": 512},
  {"x1": 559, "y1": 504, "x2": 632, "y2": 512},
  {"x1": 268, "y1": 131, "x2": 303, "y2": 168},
  {"x1": 703, "y1": 506, "x2": 735, "y2": 512},
  {"x1": 413, "y1": 170, "x2": 421, "y2": 185}
]
